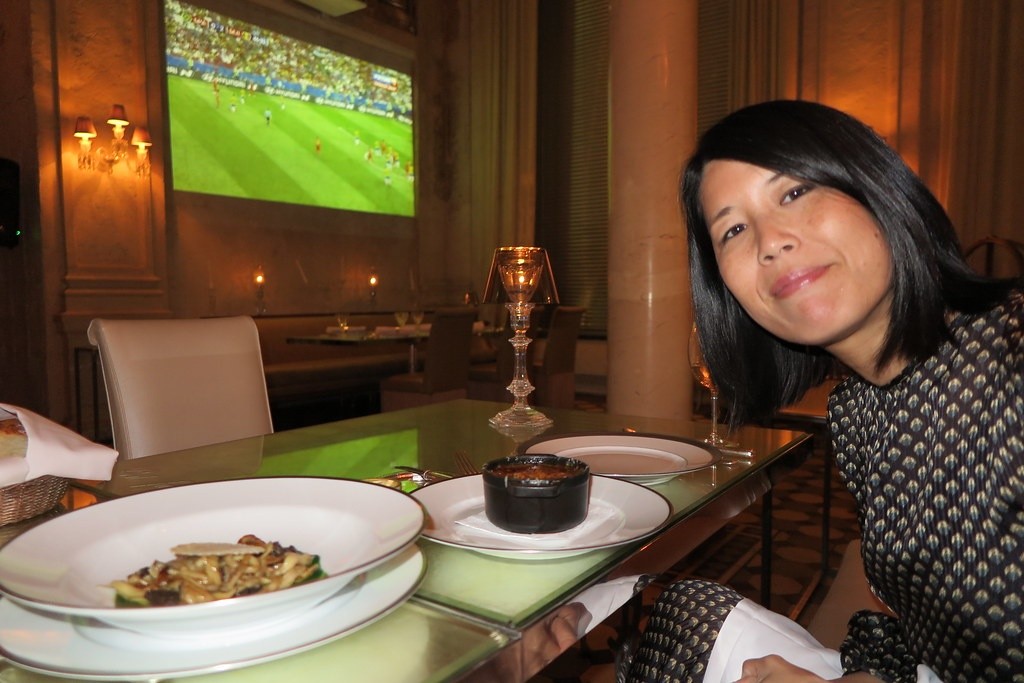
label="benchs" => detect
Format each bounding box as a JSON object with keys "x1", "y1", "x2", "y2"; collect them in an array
[{"x1": 256, "y1": 311, "x2": 432, "y2": 399}]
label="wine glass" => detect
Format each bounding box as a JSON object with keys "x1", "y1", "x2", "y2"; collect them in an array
[
  {"x1": 688, "y1": 320, "x2": 743, "y2": 449},
  {"x1": 410, "y1": 311, "x2": 425, "y2": 335},
  {"x1": 394, "y1": 311, "x2": 410, "y2": 336}
]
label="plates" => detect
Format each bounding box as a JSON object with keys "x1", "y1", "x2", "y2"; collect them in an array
[
  {"x1": 405, "y1": 470, "x2": 673, "y2": 559},
  {"x1": 1, "y1": 477, "x2": 425, "y2": 640},
  {"x1": 523, "y1": 435, "x2": 724, "y2": 487},
  {"x1": 0, "y1": 542, "x2": 429, "y2": 683}
]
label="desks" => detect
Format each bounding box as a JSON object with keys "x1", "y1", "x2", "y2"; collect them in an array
[
  {"x1": 284, "y1": 320, "x2": 543, "y2": 376},
  {"x1": 0, "y1": 395, "x2": 815, "y2": 683}
]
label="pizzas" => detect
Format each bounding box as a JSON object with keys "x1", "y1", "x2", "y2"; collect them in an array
[{"x1": 114, "y1": 534, "x2": 324, "y2": 608}]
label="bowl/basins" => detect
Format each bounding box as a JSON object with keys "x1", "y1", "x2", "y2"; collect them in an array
[{"x1": 482, "y1": 453, "x2": 592, "y2": 534}]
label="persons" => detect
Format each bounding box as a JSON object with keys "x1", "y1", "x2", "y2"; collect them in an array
[
  {"x1": 163, "y1": 1, "x2": 413, "y2": 202},
  {"x1": 621, "y1": 99, "x2": 1024, "y2": 683}
]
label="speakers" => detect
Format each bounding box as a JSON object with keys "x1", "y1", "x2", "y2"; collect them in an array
[{"x1": 0, "y1": 158, "x2": 20, "y2": 249}]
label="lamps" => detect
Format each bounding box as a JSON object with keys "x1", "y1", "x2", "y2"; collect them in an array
[{"x1": 74, "y1": 104, "x2": 151, "y2": 180}]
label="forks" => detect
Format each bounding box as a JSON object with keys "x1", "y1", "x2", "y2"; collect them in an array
[{"x1": 453, "y1": 451, "x2": 479, "y2": 477}]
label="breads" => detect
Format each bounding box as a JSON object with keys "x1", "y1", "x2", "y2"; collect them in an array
[{"x1": 0, "y1": 417, "x2": 28, "y2": 458}]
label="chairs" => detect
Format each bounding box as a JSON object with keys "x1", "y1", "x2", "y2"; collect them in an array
[
  {"x1": 87, "y1": 316, "x2": 274, "y2": 459},
  {"x1": 381, "y1": 306, "x2": 584, "y2": 410},
  {"x1": 807, "y1": 538, "x2": 899, "y2": 654}
]
